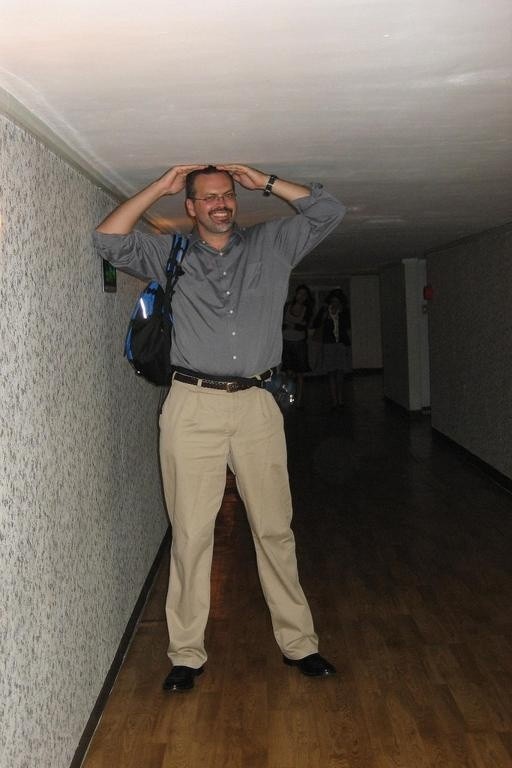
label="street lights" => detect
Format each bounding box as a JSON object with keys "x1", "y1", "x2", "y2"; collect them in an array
[
  {"x1": 312, "y1": 326, "x2": 323, "y2": 342},
  {"x1": 266, "y1": 371, "x2": 296, "y2": 409}
]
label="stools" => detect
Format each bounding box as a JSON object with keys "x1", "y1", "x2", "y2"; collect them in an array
[{"x1": 172, "y1": 367, "x2": 276, "y2": 393}]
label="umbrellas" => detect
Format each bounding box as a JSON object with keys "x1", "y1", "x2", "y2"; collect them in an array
[
  {"x1": 162, "y1": 665, "x2": 204, "y2": 690},
  {"x1": 282, "y1": 653, "x2": 336, "y2": 678}
]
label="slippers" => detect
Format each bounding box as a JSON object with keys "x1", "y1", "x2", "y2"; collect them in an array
[{"x1": 124, "y1": 232, "x2": 190, "y2": 386}]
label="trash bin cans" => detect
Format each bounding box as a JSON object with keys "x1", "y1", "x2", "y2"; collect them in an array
[{"x1": 262, "y1": 173, "x2": 278, "y2": 197}]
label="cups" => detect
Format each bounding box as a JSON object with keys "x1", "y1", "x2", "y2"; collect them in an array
[{"x1": 188, "y1": 192, "x2": 237, "y2": 204}]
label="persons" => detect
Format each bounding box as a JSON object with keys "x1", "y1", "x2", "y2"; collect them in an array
[
  {"x1": 282, "y1": 283, "x2": 317, "y2": 410},
  {"x1": 90, "y1": 160, "x2": 350, "y2": 692},
  {"x1": 308, "y1": 285, "x2": 354, "y2": 407}
]
